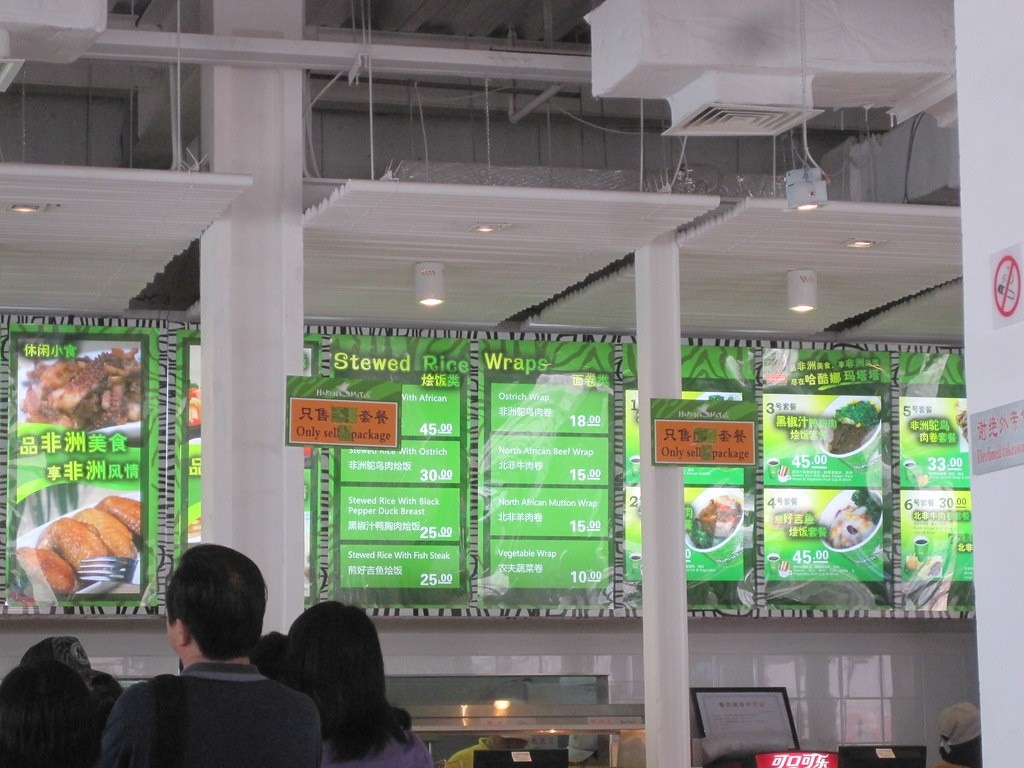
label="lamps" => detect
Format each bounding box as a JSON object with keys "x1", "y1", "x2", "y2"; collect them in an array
[
  {"x1": 414, "y1": 262, "x2": 446, "y2": 305},
  {"x1": 785, "y1": 168, "x2": 827, "y2": 209},
  {"x1": 786, "y1": 270, "x2": 818, "y2": 312}
]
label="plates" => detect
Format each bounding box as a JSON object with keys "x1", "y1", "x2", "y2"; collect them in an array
[
  {"x1": 16, "y1": 494, "x2": 140, "y2": 594},
  {"x1": 817, "y1": 395, "x2": 881, "y2": 458}
]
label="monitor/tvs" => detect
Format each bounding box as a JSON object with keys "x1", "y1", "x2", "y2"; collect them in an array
[
  {"x1": 838, "y1": 745, "x2": 926, "y2": 768},
  {"x1": 473, "y1": 749, "x2": 569, "y2": 768}
]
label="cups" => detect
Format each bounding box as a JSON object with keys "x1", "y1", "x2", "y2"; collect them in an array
[{"x1": 914, "y1": 539, "x2": 929, "y2": 562}]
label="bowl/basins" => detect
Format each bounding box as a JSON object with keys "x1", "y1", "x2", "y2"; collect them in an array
[
  {"x1": 818, "y1": 490, "x2": 883, "y2": 553},
  {"x1": 685, "y1": 488, "x2": 744, "y2": 552}
]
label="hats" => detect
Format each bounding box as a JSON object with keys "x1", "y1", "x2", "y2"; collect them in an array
[
  {"x1": 20, "y1": 636, "x2": 102, "y2": 680},
  {"x1": 937, "y1": 703, "x2": 981, "y2": 754}
]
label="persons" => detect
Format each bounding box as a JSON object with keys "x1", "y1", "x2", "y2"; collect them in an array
[
  {"x1": 284, "y1": 601, "x2": 436, "y2": 768},
  {"x1": 931, "y1": 702, "x2": 983, "y2": 768},
  {"x1": 0, "y1": 631, "x2": 122, "y2": 768},
  {"x1": 248, "y1": 631, "x2": 289, "y2": 681},
  {"x1": 444, "y1": 698, "x2": 534, "y2": 768},
  {"x1": 566, "y1": 733, "x2": 598, "y2": 766},
  {"x1": 99, "y1": 542, "x2": 324, "y2": 768}
]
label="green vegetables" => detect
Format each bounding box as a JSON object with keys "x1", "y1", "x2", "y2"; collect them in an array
[
  {"x1": 835, "y1": 400, "x2": 882, "y2": 428},
  {"x1": 690, "y1": 521, "x2": 717, "y2": 548},
  {"x1": 850, "y1": 487, "x2": 883, "y2": 524}
]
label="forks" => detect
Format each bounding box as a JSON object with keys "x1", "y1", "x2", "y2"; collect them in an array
[{"x1": 77, "y1": 556, "x2": 140, "y2": 585}]
